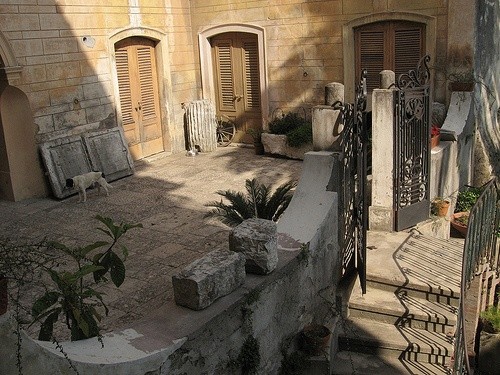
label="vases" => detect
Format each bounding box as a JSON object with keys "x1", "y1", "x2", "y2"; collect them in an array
[{"x1": 430, "y1": 133, "x2": 441, "y2": 149}]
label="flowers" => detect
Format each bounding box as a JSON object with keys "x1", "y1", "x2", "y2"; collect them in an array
[{"x1": 432, "y1": 125, "x2": 440, "y2": 137}]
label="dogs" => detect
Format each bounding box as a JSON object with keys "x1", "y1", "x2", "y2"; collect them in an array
[{"x1": 63, "y1": 171, "x2": 115, "y2": 203}]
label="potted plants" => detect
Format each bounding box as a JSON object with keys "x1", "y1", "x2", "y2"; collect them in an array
[
  {"x1": 259, "y1": 111, "x2": 312, "y2": 159},
  {"x1": 450, "y1": 71, "x2": 477, "y2": 92},
  {"x1": 479, "y1": 306, "x2": 500, "y2": 334},
  {"x1": 302, "y1": 298, "x2": 357, "y2": 375},
  {"x1": 452, "y1": 209, "x2": 470, "y2": 235},
  {"x1": 433, "y1": 194, "x2": 451, "y2": 216}
]
